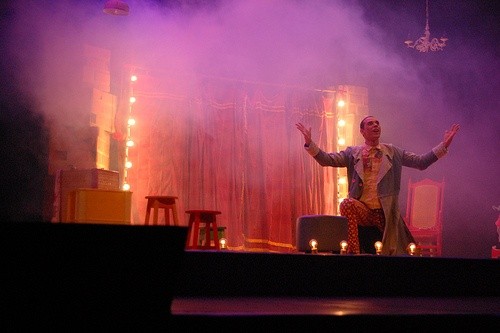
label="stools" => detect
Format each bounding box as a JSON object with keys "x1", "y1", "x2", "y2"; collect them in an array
[
  {"x1": 144, "y1": 196, "x2": 180, "y2": 226},
  {"x1": 185, "y1": 209, "x2": 222, "y2": 249}
]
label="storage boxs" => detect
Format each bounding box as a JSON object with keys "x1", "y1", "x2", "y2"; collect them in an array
[{"x1": 62, "y1": 168, "x2": 120, "y2": 190}]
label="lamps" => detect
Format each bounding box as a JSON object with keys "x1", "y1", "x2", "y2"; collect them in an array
[{"x1": 403, "y1": 0, "x2": 449, "y2": 53}]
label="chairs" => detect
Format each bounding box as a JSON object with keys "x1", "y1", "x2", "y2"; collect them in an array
[{"x1": 401, "y1": 175, "x2": 447, "y2": 257}]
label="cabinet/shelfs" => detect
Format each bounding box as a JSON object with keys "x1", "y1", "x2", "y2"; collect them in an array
[{"x1": 62, "y1": 189, "x2": 133, "y2": 224}]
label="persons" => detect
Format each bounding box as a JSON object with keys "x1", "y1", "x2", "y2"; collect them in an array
[{"x1": 295, "y1": 116, "x2": 461, "y2": 256}]
